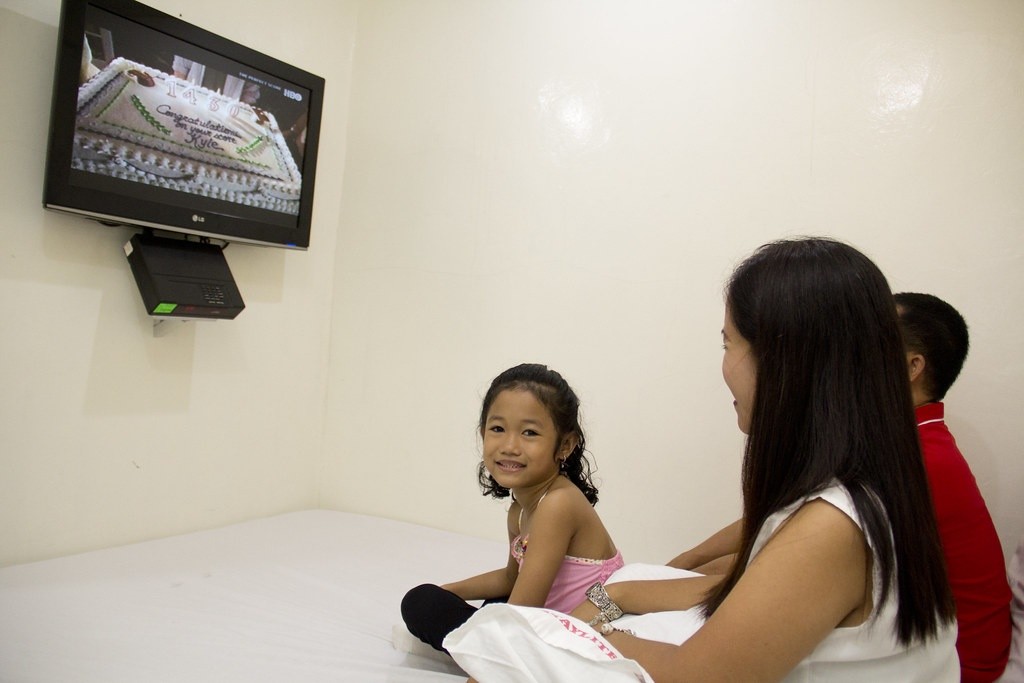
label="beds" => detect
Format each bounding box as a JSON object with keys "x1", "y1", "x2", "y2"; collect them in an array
[{"x1": 2, "y1": 493, "x2": 1024, "y2": 683}]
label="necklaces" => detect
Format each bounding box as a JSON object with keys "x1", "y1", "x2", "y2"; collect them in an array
[{"x1": 518, "y1": 490, "x2": 548, "y2": 532}]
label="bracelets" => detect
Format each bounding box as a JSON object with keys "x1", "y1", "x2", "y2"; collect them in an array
[{"x1": 584, "y1": 581, "x2": 624, "y2": 622}]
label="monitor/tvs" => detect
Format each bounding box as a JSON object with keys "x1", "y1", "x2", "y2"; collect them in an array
[{"x1": 40, "y1": 0, "x2": 325, "y2": 250}]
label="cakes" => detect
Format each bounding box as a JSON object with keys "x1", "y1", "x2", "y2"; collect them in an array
[{"x1": 73, "y1": 57, "x2": 302, "y2": 215}]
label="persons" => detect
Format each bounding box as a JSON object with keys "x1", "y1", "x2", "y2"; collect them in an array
[
  {"x1": 172, "y1": 53, "x2": 260, "y2": 104},
  {"x1": 401, "y1": 364, "x2": 624, "y2": 683},
  {"x1": 441, "y1": 240, "x2": 960, "y2": 682},
  {"x1": 666, "y1": 292, "x2": 1013, "y2": 683}
]
